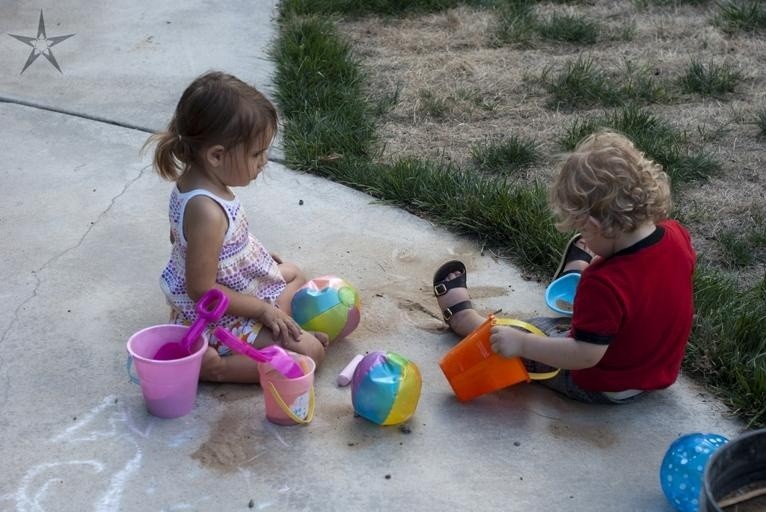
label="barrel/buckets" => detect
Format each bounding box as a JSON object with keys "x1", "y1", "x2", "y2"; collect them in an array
[
  {"x1": 438, "y1": 314, "x2": 562, "y2": 403},
  {"x1": 125, "y1": 323, "x2": 210, "y2": 420},
  {"x1": 257, "y1": 354, "x2": 317, "y2": 427}
]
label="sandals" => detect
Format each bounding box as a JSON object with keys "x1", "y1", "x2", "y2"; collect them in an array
[
  {"x1": 433, "y1": 259, "x2": 474, "y2": 325},
  {"x1": 551, "y1": 232, "x2": 593, "y2": 282}
]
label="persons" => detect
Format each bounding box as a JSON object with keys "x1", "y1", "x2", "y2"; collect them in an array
[
  {"x1": 151, "y1": 71, "x2": 329, "y2": 385},
  {"x1": 429, "y1": 131, "x2": 695, "y2": 401}
]
